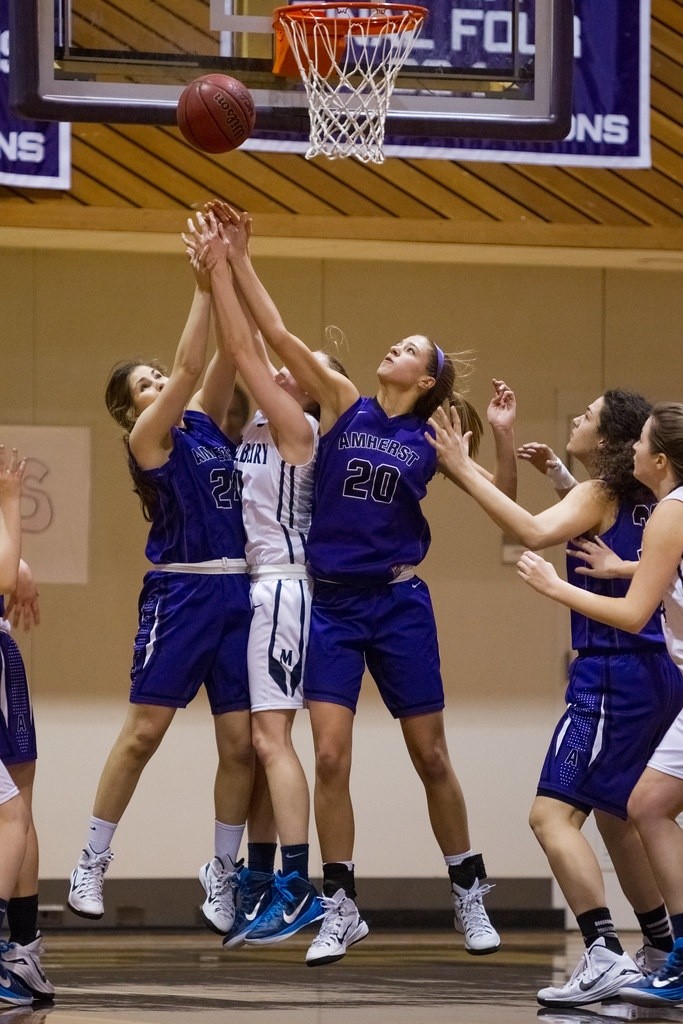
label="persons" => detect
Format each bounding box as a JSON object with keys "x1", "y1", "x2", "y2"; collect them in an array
[
  {"x1": 423, "y1": 376, "x2": 683, "y2": 1006},
  {"x1": 0, "y1": 444, "x2": 55, "y2": 1005},
  {"x1": 66, "y1": 199, "x2": 517, "y2": 968},
  {"x1": 516, "y1": 385, "x2": 683, "y2": 1009}
]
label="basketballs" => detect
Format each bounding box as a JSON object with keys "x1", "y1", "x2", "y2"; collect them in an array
[{"x1": 174, "y1": 72, "x2": 258, "y2": 157}]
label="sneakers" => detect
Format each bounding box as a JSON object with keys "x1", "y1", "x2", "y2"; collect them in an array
[
  {"x1": 632, "y1": 944, "x2": 672, "y2": 977},
  {"x1": 244, "y1": 870, "x2": 327, "y2": 945},
  {"x1": 67, "y1": 843, "x2": 114, "y2": 920},
  {"x1": 305, "y1": 889, "x2": 370, "y2": 967},
  {"x1": 0, "y1": 965, "x2": 33, "y2": 1005},
  {"x1": 619, "y1": 936, "x2": 683, "y2": 1006},
  {"x1": 450, "y1": 876, "x2": 500, "y2": 954},
  {"x1": 538, "y1": 937, "x2": 645, "y2": 1007},
  {"x1": 198, "y1": 854, "x2": 244, "y2": 936},
  {"x1": 222, "y1": 868, "x2": 276, "y2": 950},
  {"x1": 0, "y1": 930, "x2": 56, "y2": 998}
]
